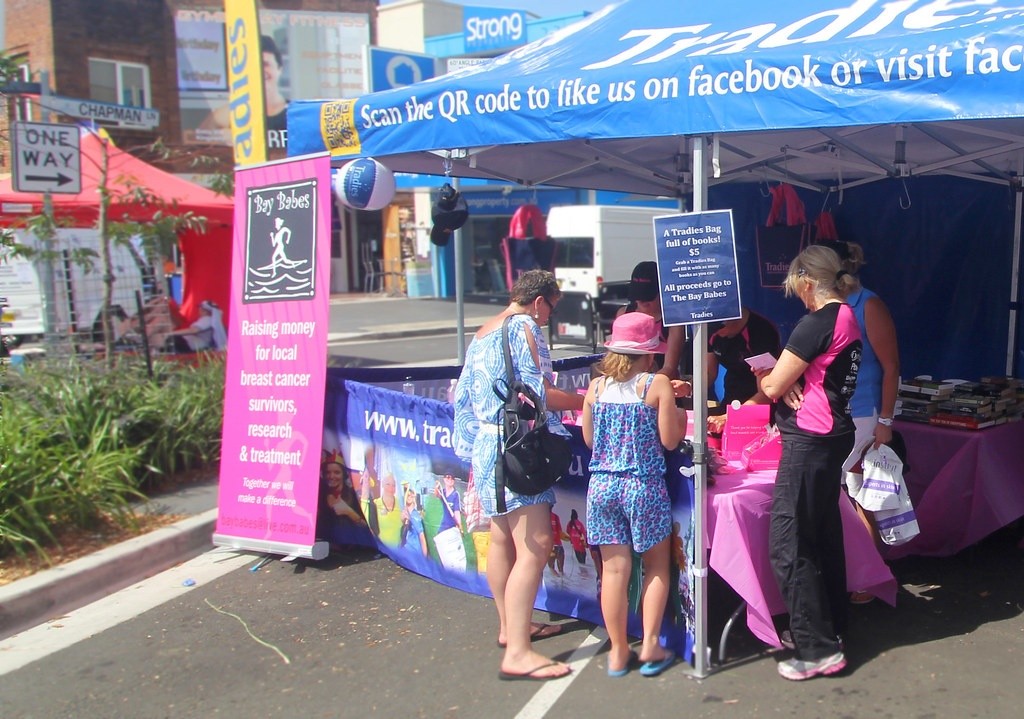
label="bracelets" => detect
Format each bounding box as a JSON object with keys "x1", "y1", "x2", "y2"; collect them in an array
[
  {"x1": 878, "y1": 417, "x2": 892, "y2": 426},
  {"x1": 685, "y1": 380, "x2": 693, "y2": 398}
]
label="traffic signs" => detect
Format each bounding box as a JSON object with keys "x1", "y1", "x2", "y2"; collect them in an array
[{"x1": 11, "y1": 119, "x2": 82, "y2": 194}]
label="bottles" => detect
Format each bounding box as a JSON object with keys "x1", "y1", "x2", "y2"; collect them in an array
[
  {"x1": 447, "y1": 378, "x2": 457, "y2": 403},
  {"x1": 403, "y1": 376, "x2": 415, "y2": 395}
]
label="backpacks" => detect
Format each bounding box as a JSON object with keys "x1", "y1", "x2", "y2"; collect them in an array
[{"x1": 492, "y1": 313, "x2": 572, "y2": 513}]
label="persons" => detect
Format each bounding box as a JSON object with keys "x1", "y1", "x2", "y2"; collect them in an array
[
  {"x1": 614, "y1": 262, "x2": 683, "y2": 409},
  {"x1": 751, "y1": 239, "x2": 901, "y2": 603},
  {"x1": 241, "y1": 33, "x2": 298, "y2": 151},
  {"x1": 454, "y1": 269, "x2": 585, "y2": 679},
  {"x1": 95, "y1": 296, "x2": 226, "y2": 354},
  {"x1": 757, "y1": 246, "x2": 862, "y2": 681},
  {"x1": 670, "y1": 303, "x2": 780, "y2": 418},
  {"x1": 582, "y1": 311, "x2": 687, "y2": 677}
]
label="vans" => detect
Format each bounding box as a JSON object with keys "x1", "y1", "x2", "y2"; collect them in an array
[{"x1": 545, "y1": 204, "x2": 685, "y2": 339}]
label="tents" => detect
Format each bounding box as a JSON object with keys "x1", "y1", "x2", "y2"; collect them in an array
[
  {"x1": 0, "y1": 133, "x2": 235, "y2": 366},
  {"x1": 283, "y1": 0, "x2": 1024, "y2": 678}
]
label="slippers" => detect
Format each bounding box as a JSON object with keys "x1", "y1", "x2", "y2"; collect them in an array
[
  {"x1": 606, "y1": 643, "x2": 629, "y2": 676},
  {"x1": 640, "y1": 648, "x2": 678, "y2": 677},
  {"x1": 498, "y1": 659, "x2": 571, "y2": 681},
  {"x1": 496, "y1": 623, "x2": 563, "y2": 647}
]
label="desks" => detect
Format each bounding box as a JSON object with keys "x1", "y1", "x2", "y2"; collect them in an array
[
  {"x1": 883, "y1": 409, "x2": 1024, "y2": 560},
  {"x1": 529, "y1": 397, "x2": 898, "y2": 664}
]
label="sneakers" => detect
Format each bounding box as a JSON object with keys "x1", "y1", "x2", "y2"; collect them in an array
[
  {"x1": 781, "y1": 628, "x2": 844, "y2": 650},
  {"x1": 777, "y1": 650, "x2": 848, "y2": 680}
]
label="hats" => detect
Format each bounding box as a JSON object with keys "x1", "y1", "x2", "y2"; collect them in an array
[
  {"x1": 430, "y1": 194, "x2": 470, "y2": 247},
  {"x1": 604, "y1": 312, "x2": 669, "y2": 354},
  {"x1": 625, "y1": 261, "x2": 659, "y2": 301}
]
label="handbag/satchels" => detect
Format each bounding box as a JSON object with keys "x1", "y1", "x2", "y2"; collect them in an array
[
  {"x1": 854, "y1": 442, "x2": 921, "y2": 545},
  {"x1": 755, "y1": 182, "x2": 848, "y2": 288},
  {"x1": 501, "y1": 201, "x2": 560, "y2": 294}
]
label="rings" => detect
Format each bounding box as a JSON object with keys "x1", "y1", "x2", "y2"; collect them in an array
[{"x1": 789, "y1": 392, "x2": 795, "y2": 396}]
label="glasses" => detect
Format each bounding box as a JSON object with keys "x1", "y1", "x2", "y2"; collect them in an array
[{"x1": 533, "y1": 294, "x2": 558, "y2": 317}]
